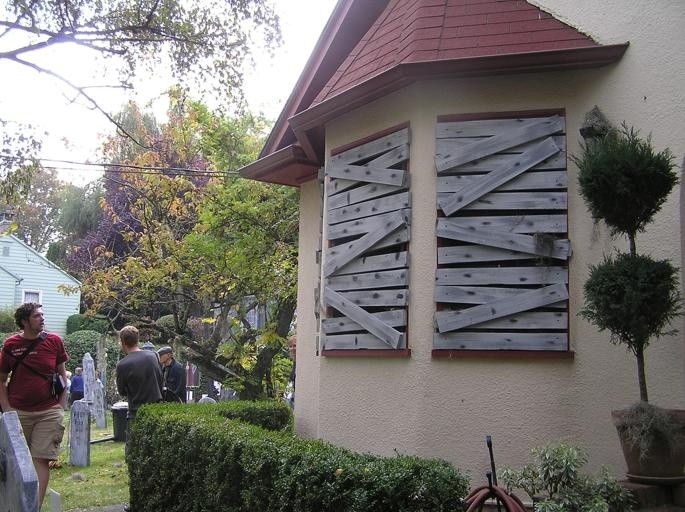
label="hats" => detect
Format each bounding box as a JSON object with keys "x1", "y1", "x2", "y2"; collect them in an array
[{"x1": 156, "y1": 346, "x2": 173, "y2": 355}]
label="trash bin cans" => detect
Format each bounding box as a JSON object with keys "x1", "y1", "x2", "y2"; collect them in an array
[{"x1": 111, "y1": 402, "x2": 129, "y2": 442}]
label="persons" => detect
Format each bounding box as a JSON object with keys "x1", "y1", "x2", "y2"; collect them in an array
[
  {"x1": 114, "y1": 325, "x2": 165, "y2": 417},
  {"x1": 156, "y1": 347, "x2": 186, "y2": 403},
  {"x1": 287, "y1": 335, "x2": 296, "y2": 391},
  {"x1": 51, "y1": 366, "x2": 105, "y2": 413},
  {"x1": 0, "y1": 302, "x2": 70, "y2": 512}
]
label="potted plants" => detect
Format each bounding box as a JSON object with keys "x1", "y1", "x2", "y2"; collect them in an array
[{"x1": 561, "y1": 116, "x2": 685, "y2": 487}]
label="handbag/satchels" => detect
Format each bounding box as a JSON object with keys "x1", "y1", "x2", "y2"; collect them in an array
[
  {"x1": 49, "y1": 372, "x2": 65, "y2": 397},
  {"x1": 162, "y1": 386, "x2": 182, "y2": 404}
]
